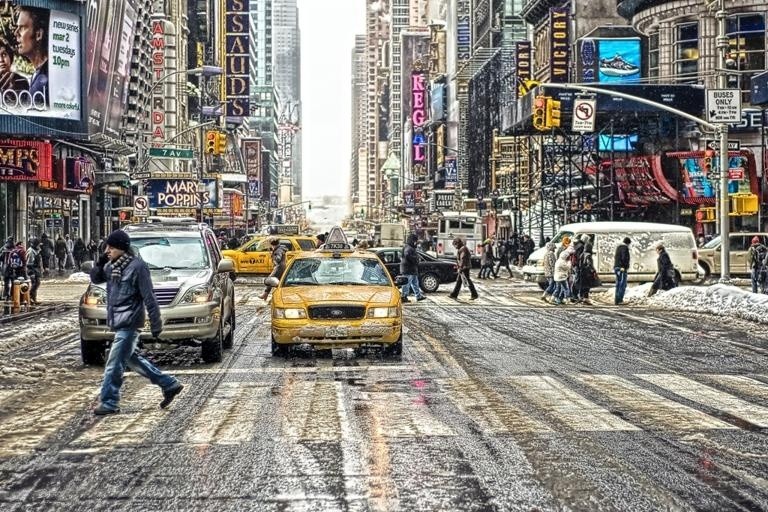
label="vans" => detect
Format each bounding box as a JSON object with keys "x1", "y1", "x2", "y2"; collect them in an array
[{"x1": 518, "y1": 220, "x2": 707, "y2": 294}]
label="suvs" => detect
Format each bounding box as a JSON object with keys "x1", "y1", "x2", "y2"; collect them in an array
[{"x1": 694, "y1": 231, "x2": 768, "y2": 279}]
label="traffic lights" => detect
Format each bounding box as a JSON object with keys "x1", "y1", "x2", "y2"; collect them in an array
[
  {"x1": 533, "y1": 95, "x2": 546, "y2": 130},
  {"x1": 704, "y1": 150, "x2": 715, "y2": 174},
  {"x1": 218, "y1": 134, "x2": 227, "y2": 154},
  {"x1": 550, "y1": 98, "x2": 563, "y2": 129},
  {"x1": 207, "y1": 131, "x2": 219, "y2": 157},
  {"x1": 695, "y1": 208, "x2": 710, "y2": 224}
]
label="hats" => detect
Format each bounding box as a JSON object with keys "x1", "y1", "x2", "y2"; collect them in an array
[
  {"x1": 623, "y1": 237, "x2": 629, "y2": 243},
  {"x1": 103, "y1": 230, "x2": 131, "y2": 251},
  {"x1": 656, "y1": 243, "x2": 665, "y2": 250},
  {"x1": 750, "y1": 236, "x2": 759, "y2": 245}
]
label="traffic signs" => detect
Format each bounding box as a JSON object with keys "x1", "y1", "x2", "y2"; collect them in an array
[
  {"x1": 130, "y1": 170, "x2": 155, "y2": 183},
  {"x1": 150, "y1": 147, "x2": 196, "y2": 159},
  {"x1": 707, "y1": 88, "x2": 741, "y2": 124},
  {"x1": 704, "y1": 138, "x2": 742, "y2": 153},
  {"x1": 706, "y1": 168, "x2": 746, "y2": 183}
]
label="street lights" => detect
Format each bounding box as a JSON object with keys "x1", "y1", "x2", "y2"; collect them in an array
[
  {"x1": 133, "y1": 61, "x2": 230, "y2": 205},
  {"x1": 419, "y1": 140, "x2": 460, "y2": 154},
  {"x1": 380, "y1": 173, "x2": 414, "y2": 182},
  {"x1": 145, "y1": 112, "x2": 245, "y2": 175}
]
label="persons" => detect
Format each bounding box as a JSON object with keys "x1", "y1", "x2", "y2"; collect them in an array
[
  {"x1": 613, "y1": 237, "x2": 631, "y2": 306},
  {"x1": 600, "y1": 55, "x2": 629, "y2": 70},
  {"x1": 648, "y1": 245, "x2": 671, "y2": 297},
  {"x1": 540, "y1": 234, "x2": 595, "y2": 306},
  {"x1": 748, "y1": 236, "x2": 768, "y2": 293},
  {"x1": 400, "y1": 235, "x2": 427, "y2": 303},
  {"x1": 696, "y1": 232, "x2": 707, "y2": 246},
  {"x1": 13, "y1": 7, "x2": 48, "y2": 101},
  {"x1": 89, "y1": 229, "x2": 185, "y2": 416},
  {"x1": 419, "y1": 233, "x2": 550, "y2": 280},
  {"x1": 447, "y1": 237, "x2": 478, "y2": 300},
  {"x1": 0, "y1": 42, "x2": 29, "y2": 97}
]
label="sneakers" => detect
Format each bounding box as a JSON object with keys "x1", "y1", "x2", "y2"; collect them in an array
[
  {"x1": 401, "y1": 295, "x2": 409, "y2": 302},
  {"x1": 448, "y1": 294, "x2": 457, "y2": 300},
  {"x1": 418, "y1": 296, "x2": 428, "y2": 301},
  {"x1": 469, "y1": 295, "x2": 478, "y2": 300},
  {"x1": 159, "y1": 379, "x2": 185, "y2": 409},
  {"x1": 93, "y1": 402, "x2": 120, "y2": 416},
  {"x1": 1, "y1": 291, "x2": 41, "y2": 306},
  {"x1": 37, "y1": 264, "x2": 82, "y2": 275},
  {"x1": 614, "y1": 299, "x2": 629, "y2": 306},
  {"x1": 540, "y1": 296, "x2": 594, "y2": 307},
  {"x1": 477, "y1": 274, "x2": 514, "y2": 280}
]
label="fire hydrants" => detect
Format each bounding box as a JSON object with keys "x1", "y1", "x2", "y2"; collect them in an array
[{"x1": 12, "y1": 275, "x2": 32, "y2": 316}]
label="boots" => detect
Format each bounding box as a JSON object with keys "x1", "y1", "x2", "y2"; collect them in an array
[{"x1": 257, "y1": 291, "x2": 269, "y2": 301}]
